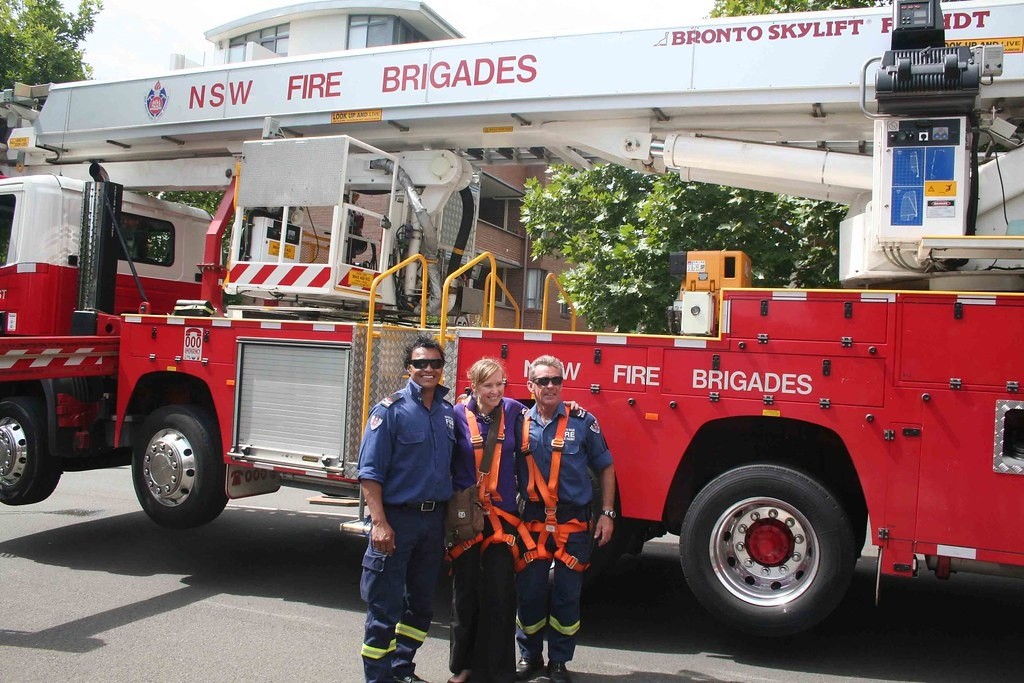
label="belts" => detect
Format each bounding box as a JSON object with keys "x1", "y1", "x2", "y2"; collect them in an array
[{"x1": 397, "y1": 500, "x2": 448, "y2": 511}]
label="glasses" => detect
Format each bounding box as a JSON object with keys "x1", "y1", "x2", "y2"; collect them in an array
[
  {"x1": 405, "y1": 359, "x2": 445, "y2": 369},
  {"x1": 530, "y1": 376, "x2": 563, "y2": 386}
]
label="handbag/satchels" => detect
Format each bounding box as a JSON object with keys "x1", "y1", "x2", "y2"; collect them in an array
[{"x1": 445, "y1": 484, "x2": 484, "y2": 550}]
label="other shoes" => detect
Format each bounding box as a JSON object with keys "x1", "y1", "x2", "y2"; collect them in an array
[
  {"x1": 448, "y1": 667, "x2": 474, "y2": 683},
  {"x1": 392, "y1": 674, "x2": 429, "y2": 683}
]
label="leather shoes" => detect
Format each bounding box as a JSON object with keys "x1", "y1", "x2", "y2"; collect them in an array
[
  {"x1": 545, "y1": 660, "x2": 572, "y2": 683},
  {"x1": 515, "y1": 655, "x2": 544, "y2": 681}
]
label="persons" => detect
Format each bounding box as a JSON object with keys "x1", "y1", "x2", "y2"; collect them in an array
[
  {"x1": 514, "y1": 355, "x2": 616, "y2": 683},
  {"x1": 356, "y1": 335, "x2": 454, "y2": 683},
  {"x1": 447, "y1": 358, "x2": 587, "y2": 683}
]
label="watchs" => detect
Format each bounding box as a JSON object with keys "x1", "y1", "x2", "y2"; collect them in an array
[{"x1": 599, "y1": 509, "x2": 616, "y2": 519}]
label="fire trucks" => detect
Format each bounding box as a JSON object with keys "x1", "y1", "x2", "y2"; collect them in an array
[{"x1": 0, "y1": 0, "x2": 1024, "y2": 642}]
